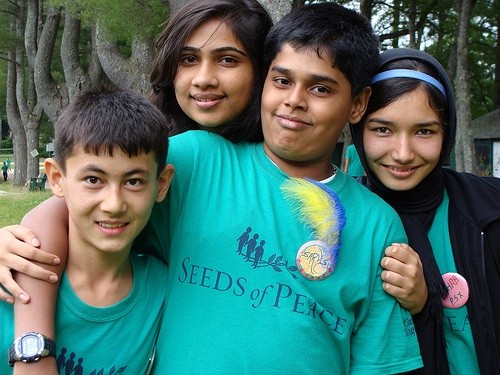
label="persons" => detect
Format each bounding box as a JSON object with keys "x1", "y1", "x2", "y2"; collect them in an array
[
  {"x1": 1, "y1": 0, "x2": 274, "y2": 305},
  {"x1": 0, "y1": 85, "x2": 174, "y2": 375},
  {"x1": 9, "y1": 0, "x2": 427, "y2": 375},
  {"x1": 7, "y1": 157, "x2": 12, "y2": 171},
  {"x1": 345, "y1": 47, "x2": 500, "y2": 374},
  {"x1": 345, "y1": 141, "x2": 367, "y2": 186},
  {"x1": 2, "y1": 161, "x2": 8, "y2": 181}
]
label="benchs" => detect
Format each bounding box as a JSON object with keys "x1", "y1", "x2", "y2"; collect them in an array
[{"x1": 30, "y1": 173, "x2": 47, "y2": 191}]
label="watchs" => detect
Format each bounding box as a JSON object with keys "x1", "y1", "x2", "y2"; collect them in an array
[{"x1": 8, "y1": 331, "x2": 57, "y2": 366}]
